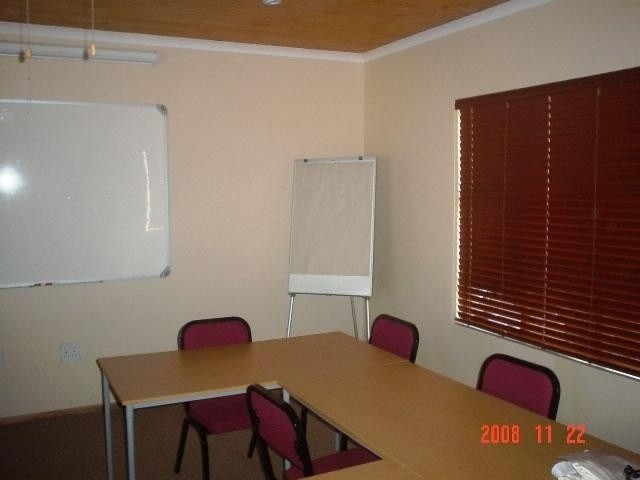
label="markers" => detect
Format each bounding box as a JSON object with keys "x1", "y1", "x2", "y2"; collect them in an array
[{"x1": 34, "y1": 283, "x2": 52, "y2": 286}]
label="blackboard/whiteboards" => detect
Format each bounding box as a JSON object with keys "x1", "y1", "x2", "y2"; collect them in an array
[{"x1": 0, "y1": 99, "x2": 172, "y2": 289}]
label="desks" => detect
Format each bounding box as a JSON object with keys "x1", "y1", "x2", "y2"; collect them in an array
[{"x1": 95, "y1": 331, "x2": 639, "y2": 480}]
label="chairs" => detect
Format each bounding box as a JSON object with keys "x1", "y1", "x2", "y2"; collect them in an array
[
  {"x1": 477, "y1": 354, "x2": 560, "y2": 421},
  {"x1": 245, "y1": 382, "x2": 381, "y2": 478},
  {"x1": 175, "y1": 315, "x2": 260, "y2": 479},
  {"x1": 289, "y1": 313, "x2": 419, "y2": 452}
]
